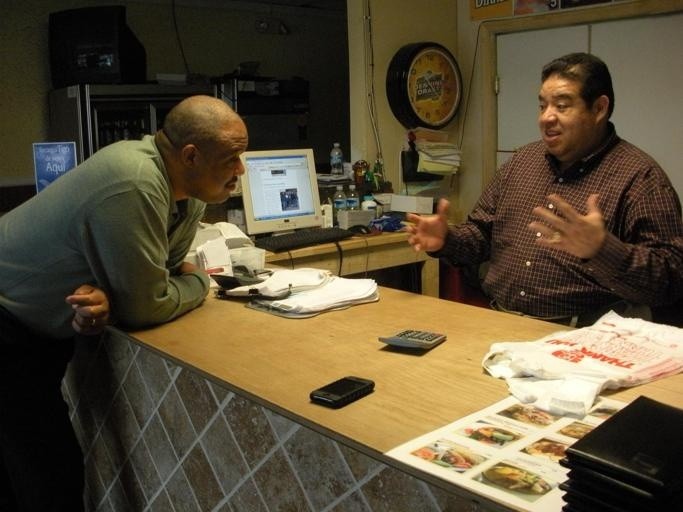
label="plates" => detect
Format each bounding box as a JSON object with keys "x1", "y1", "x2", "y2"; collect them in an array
[
  {"x1": 465, "y1": 395, "x2": 614, "y2": 460},
  {"x1": 415, "y1": 441, "x2": 485, "y2": 472}
]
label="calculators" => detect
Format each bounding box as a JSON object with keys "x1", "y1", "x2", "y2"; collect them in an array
[{"x1": 378, "y1": 329, "x2": 446, "y2": 349}]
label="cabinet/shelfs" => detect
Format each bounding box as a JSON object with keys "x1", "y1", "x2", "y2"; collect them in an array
[{"x1": 492, "y1": 12, "x2": 681, "y2": 209}]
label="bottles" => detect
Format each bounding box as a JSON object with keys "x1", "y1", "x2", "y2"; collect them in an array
[
  {"x1": 347, "y1": 184, "x2": 361, "y2": 211},
  {"x1": 328, "y1": 141, "x2": 342, "y2": 175},
  {"x1": 99, "y1": 116, "x2": 165, "y2": 147},
  {"x1": 362, "y1": 196, "x2": 376, "y2": 211},
  {"x1": 357, "y1": 159, "x2": 382, "y2": 184},
  {"x1": 334, "y1": 185, "x2": 347, "y2": 216}
]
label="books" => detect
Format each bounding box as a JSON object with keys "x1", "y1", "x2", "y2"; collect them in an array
[{"x1": 559, "y1": 396, "x2": 683, "y2": 512}]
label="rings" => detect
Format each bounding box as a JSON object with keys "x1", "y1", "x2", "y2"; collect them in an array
[
  {"x1": 91, "y1": 319, "x2": 95, "y2": 327},
  {"x1": 553, "y1": 231, "x2": 561, "y2": 244}
]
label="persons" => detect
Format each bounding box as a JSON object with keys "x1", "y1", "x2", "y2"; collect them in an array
[
  {"x1": 0, "y1": 94, "x2": 248, "y2": 512},
  {"x1": 406, "y1": 53, "x2": 683, "y2": 327}
]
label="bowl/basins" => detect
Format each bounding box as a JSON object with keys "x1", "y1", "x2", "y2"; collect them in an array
[{"x1": 481, "y1": 465, "x2": 551, "y2": 499}]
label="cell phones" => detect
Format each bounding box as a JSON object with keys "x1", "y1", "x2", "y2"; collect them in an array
[{"x1": 309, "y1": 375, "x2": 375, "y2": 410}]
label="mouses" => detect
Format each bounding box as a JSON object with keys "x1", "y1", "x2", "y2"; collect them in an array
[{"x1": 348, "y1": 224, "x2": 372, "y2": 235}]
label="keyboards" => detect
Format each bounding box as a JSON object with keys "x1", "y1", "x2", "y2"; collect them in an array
[{"x1": 255, "y1": 227, "x2": 354, "y2": 253}]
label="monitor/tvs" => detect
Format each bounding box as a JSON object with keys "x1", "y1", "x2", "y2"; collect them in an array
[
  {"x1": 238, "y1": 148, "x2": 323, "y2": 236},
  {"x1": 49, "y1": 5, "x2": 147, "y2": 85}
]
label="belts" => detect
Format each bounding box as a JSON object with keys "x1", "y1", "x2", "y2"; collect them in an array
[{"x1": 489, "y1": 299, "x2": 639, "y2": 329}]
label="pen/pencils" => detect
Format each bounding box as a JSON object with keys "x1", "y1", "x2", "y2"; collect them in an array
[{"x1": 205, "y1": 269, "x2": 224, "y2": 273}]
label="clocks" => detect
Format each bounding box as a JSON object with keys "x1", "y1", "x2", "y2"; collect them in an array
[{"x1": 384, "y1": 38, "x2": 461, "y2": 129}]
